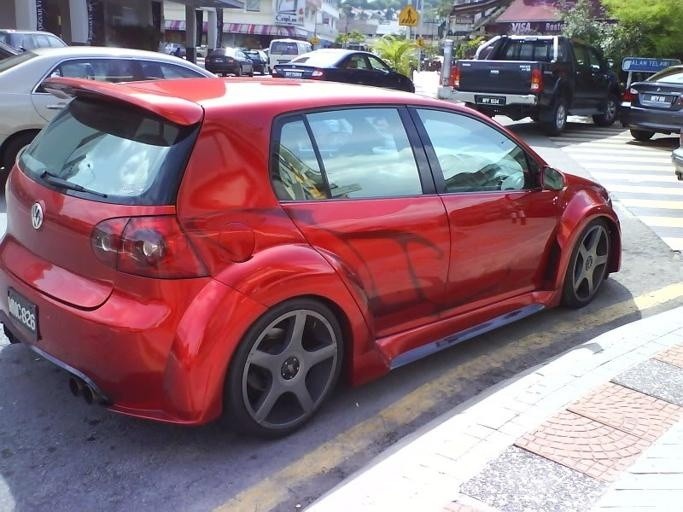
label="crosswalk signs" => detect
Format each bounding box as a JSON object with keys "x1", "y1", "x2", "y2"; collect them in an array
[{"x1": 399, "y1": 5, "x2": 418, "y2": 26}]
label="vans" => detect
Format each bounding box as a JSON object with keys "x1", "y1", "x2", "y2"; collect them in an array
[{"x1": 268, "y1": 38, "x2": 313, "y2": 74}]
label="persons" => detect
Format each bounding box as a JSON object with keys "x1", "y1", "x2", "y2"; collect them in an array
[
  {"x1": 436, "y1": 61, "x2": 441, "y2": 71},
  {"x1": 430, "y1": 61, "x2": 435, "y2": 71},
  {"x1": 174, "y1": 48, "x2": 182, "y2": 58}
]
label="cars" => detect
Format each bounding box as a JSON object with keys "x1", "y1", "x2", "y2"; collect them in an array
[
  {"x1": 205, "y1": 47, "x2": 269, "y2": 78},
  {"x1": 0, "y1": 29, "x2": 220, "y2": 194},
  {"x1": 0, "y1": 78, "x2": 621, "y2": 440},
  {"x1": 620, "y1": 64, "x2": 683, "y2": 139},
  {"x1": 272, "y1": 47, "x2": 415, "y2": 93}
]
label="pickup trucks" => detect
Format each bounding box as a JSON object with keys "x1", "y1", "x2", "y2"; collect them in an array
[{"x1": 450, "y1": 34, "x2": 623, "y2": 136}]
